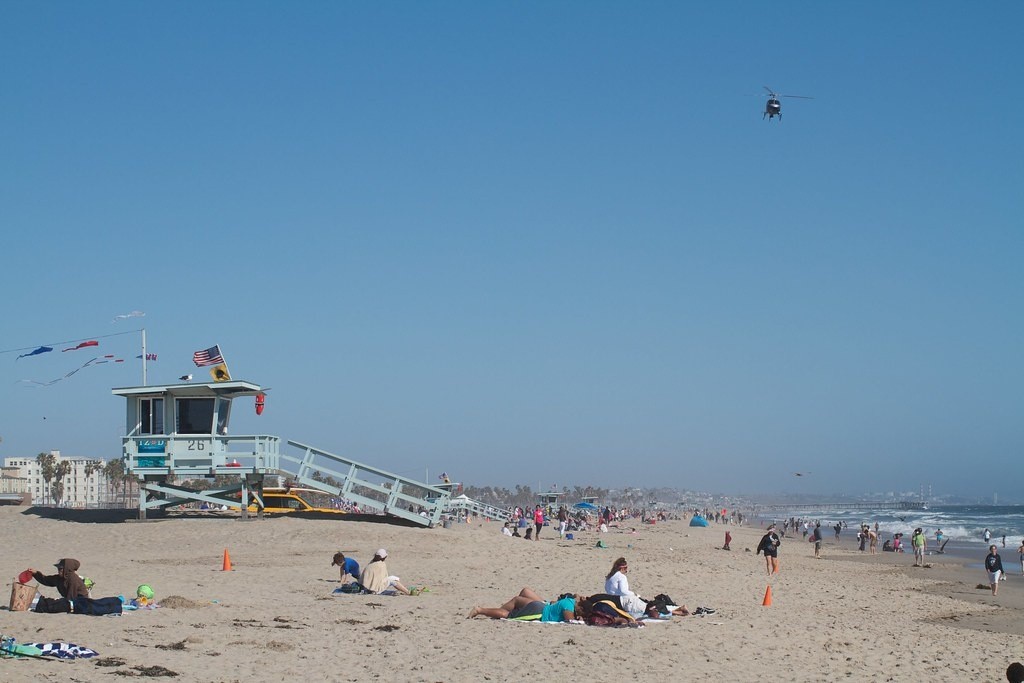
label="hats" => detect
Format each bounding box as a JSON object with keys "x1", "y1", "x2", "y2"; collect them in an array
[
  {"x1": 54, "y1": 560, "x2": 65, "y2": 569},
  {"x1": 768, "y1": 528, "x2": 776, "y2": 532},
  {"x1": 375, "y1": 548, "x2": 388, "y2": 558}
]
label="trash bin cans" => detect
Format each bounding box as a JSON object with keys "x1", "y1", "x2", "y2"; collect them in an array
[
  {"x1": 458, "y1": 517, "x2": 462, "y2": 524},
  {"x1": 443, "y1": 520, "x2": 451, "y2": 529}
]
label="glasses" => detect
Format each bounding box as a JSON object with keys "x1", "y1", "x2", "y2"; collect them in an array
[{"x1": 58, "y1": 568, "x2": 64, "y2": 571}]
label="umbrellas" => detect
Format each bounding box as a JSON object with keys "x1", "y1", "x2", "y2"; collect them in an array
[{"x1": 572, "y1": 502, "x2": 597, "y2": 509}]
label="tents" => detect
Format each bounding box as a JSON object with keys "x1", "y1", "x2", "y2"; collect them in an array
[{"x1": 689, "y1": 516, "x2": 709, "y2": 527}]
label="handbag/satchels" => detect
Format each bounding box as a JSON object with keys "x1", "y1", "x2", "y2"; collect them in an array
[{"x1": 9, "y1": 576, "x2": 39, "y2": 612}]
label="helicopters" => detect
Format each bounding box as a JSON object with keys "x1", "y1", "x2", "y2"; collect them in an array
[{"x1": 738, "y1": 86, "x2": 817, "y2": 123}]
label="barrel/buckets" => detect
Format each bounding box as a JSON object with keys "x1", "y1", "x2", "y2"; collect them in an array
[{"x1": 10, "y1": 577, "x2": 39, "y2": 612}]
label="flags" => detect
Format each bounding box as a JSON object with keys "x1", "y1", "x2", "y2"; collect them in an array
[
  {"x1": 210, "y1": 363, "x2": 230, "y2": 382},
  {"x1": 192, "y1": 345, "x2": 224, "y2": 367}
]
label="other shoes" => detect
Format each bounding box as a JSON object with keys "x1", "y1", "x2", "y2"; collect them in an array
[
  {"x1": 999, "y1": 575, "x2": 1007, "y2": 581},
  {"x1": 696, "y1": 606, "x2": 715, "y2": 615}
]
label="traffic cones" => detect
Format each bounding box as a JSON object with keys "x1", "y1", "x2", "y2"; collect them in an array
[
  {"x1": 762, "y1": 586, "x2": 772, "y2": 606},
  {"x1": 219, "y1": 548, "x2": 236, "y2": 571}
]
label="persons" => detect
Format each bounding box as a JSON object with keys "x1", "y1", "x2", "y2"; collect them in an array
[
  {"x1": 30, "y1": 590, "x2": 137, "y2": 615},
  {"x1": 1002, "y1": 535, "x2": 1006, "y2": 548},
  {"x1": 331, "y1": 553, "x2": 360, "y2": 586},
  {"x1": 1017, "y1": 540, "x2": 1024, "y2": 576},
  {"x1": 934, "y1": 529, "x2": 943, "y2": 545},
  {"x1": 813, "y1": 523, "x2": 822, "y2": 559},
  {"x1": 469, "y1": 588, "x2": 692, "y2": 622},
  {"x1": 335, "y1": 496, "x2": 360, "y2": 514},
  {"x1": 910, "y1": 527, "x2": 928, "y2": 565},
  {"x1": 762, "y1": 525, "x2": 780, "y2": 576},
  {"x1": 408, "y1": 504, "x2": 422, "y2": 514},
  {"x1": 359, "y1": 549, "x2": 422, "y2": 595},
  {"x1": 984, "y1": 529, "x2": 991, "y2": 543},
  {"x1": 834, "y1": 520, "x2": 847, "y2": 540},
  {"x1": 984, "y1": 545, "x2": 1004, "y2": 596},
  {"x1": 604, "y1": 558, "x2": 634, "y2": 596},
  {"x1": 471, "y1": 480, "x2": 695, "y2": 544},
  {"x1": 773, "y1": 516, "x2": 819, "y2": 541},
  {"x1": 883, "y1": 532, "x2": 904, "y2": 553},
  {"x1": 857, "y1": 522, "x2": 882, "y2": 554},
  {"x1": 28, "y1": 559, "x2": 89, "y2": 598},
  {"x1": 693, "y1": 491, "x2": 759, "y2": 527}
]
